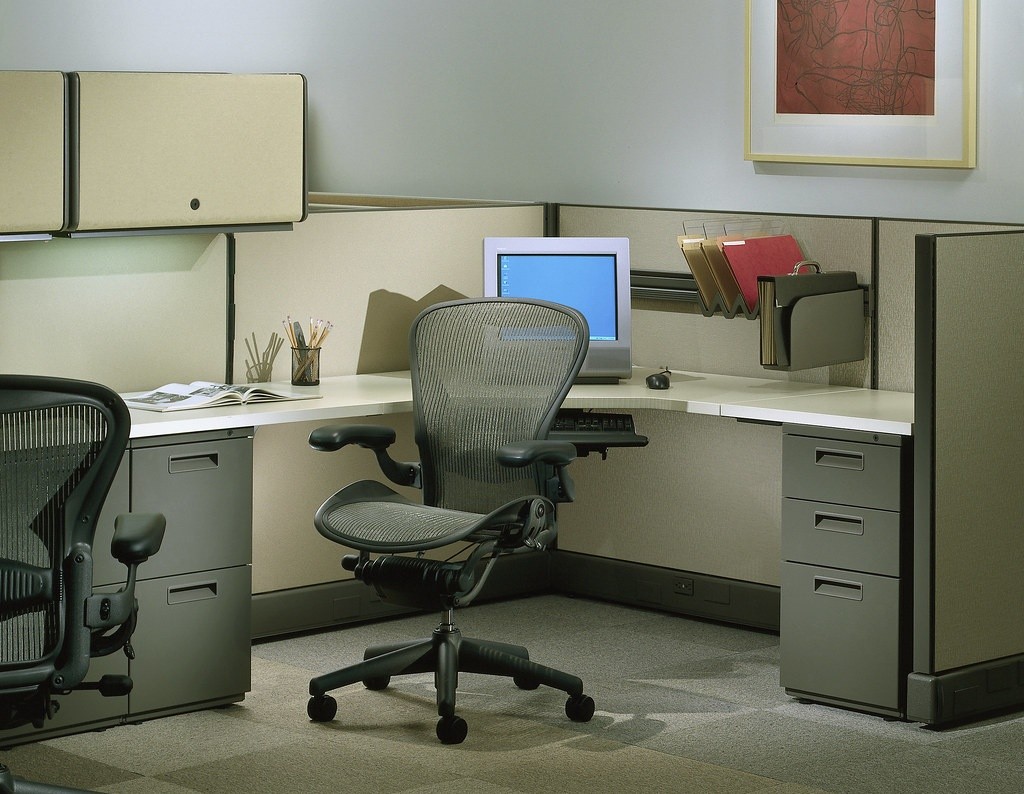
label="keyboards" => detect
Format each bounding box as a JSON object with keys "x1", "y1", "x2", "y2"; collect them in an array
[{"x1": 548, "y1": 411, "x2": 636, "y2": 433}]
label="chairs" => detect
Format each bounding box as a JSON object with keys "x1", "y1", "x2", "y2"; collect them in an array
[
  {"x1": 0, "y1": 373, "x2": 166, "y2": 794},
  {"x1": 305, "y1": 297, "x2": 598, "y2": 747}
]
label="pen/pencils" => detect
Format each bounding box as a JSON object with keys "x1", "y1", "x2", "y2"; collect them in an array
[{"x1": 283, "y1": 314, "x2": 333, "y2": 382}]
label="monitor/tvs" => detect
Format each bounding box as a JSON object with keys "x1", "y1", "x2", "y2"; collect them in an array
[{"x1": 481, "y1": 236, "x2": 633, "y2": 385}]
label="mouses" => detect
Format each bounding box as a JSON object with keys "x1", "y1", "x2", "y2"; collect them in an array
[{"x1": 646, "y1": 374, "x2": 670, "y2": 389}]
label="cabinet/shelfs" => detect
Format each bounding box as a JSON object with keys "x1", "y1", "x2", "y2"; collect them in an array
[
  {"x1": 0, "y1": 426, "x2": 257, "y2": 750},
  {"x1": 775, "y1": 423, "x2": 915, "y2": 722}
]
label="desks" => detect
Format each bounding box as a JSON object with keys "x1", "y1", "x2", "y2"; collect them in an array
[{"x1": 1, "y1": 355, "x2": 916, "y2": 748}]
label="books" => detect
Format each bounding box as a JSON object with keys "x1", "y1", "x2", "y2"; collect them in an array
[{"x1": 122, "y1": 381, "x2": 325, "y2": 415}]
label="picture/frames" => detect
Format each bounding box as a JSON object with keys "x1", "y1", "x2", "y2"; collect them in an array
[{"x1": 741, "y1": 1, "x2": 982, "y2": 171}]
